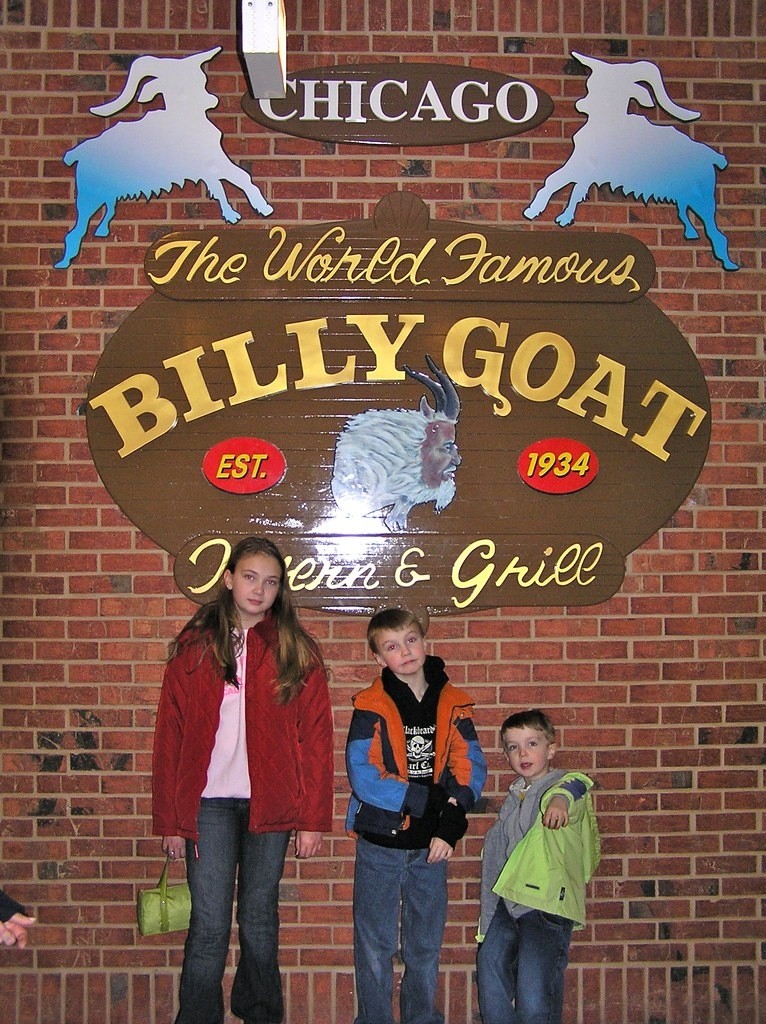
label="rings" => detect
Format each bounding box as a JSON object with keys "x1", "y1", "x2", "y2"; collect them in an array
[{"x1": 169, "y1": 851, "x2": 174, "y2": 855}]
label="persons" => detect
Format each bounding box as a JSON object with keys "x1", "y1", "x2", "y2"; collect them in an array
[
  {"x1": 476, "y1": 707, "x2": 601, "y2": 1024},
  {"x1": 0, "y1": 889, "x2": 36, "y2": 951},
  {"x1": 345, "y1": 609, "x2": 489, "y2": 1024},
  {"x1": 148, "y1": 537, "x2": 334, "y2": 1024}
]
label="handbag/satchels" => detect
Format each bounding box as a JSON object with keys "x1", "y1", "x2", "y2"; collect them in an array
[{"x1": 136, "y1": 857, "x2": 192, "y2": 936}]
